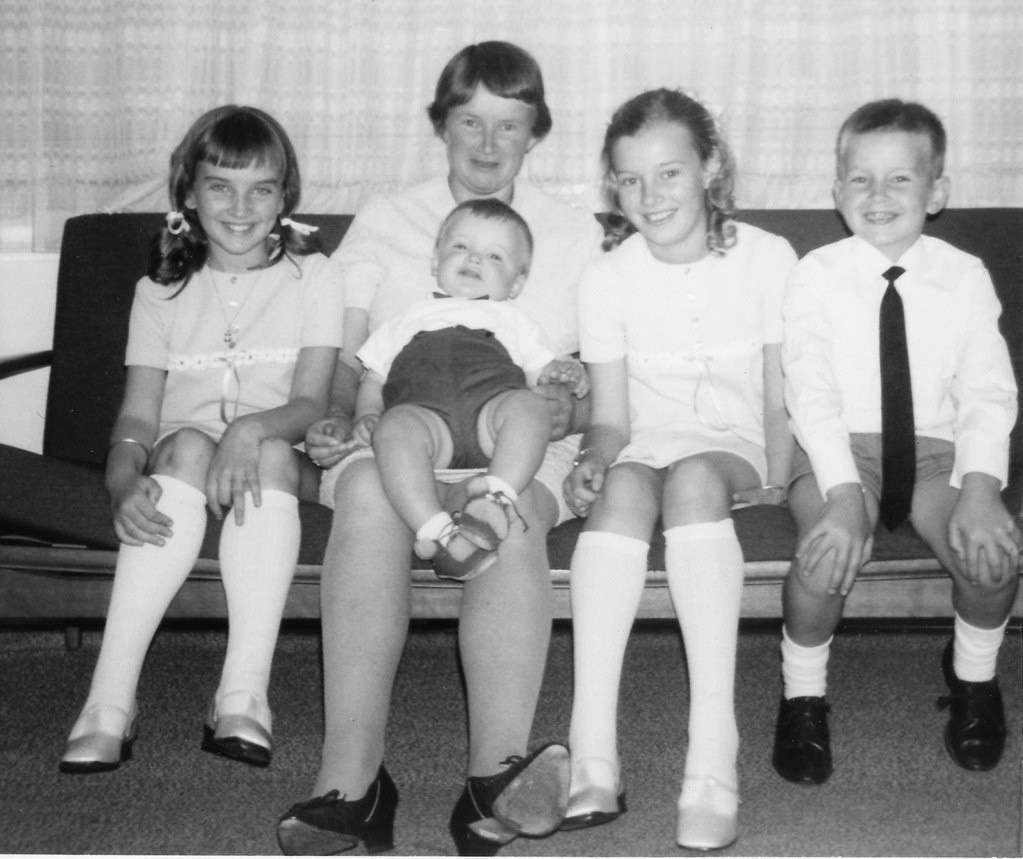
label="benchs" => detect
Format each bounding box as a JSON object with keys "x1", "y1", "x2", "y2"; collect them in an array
[{"x1": 0, "y1": 207, "x2": 1023, "y2": 650}]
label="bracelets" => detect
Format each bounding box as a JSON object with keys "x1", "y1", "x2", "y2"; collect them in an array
[
  {"x1": 574, "y1": 448, "x2": 591, "y2": 463},
  {"x1": 760, "y1": 484, "x2": 785, "y2": 491},
  {"x1": 109, "y1": 438, "x2": 151, "y2": 461}
]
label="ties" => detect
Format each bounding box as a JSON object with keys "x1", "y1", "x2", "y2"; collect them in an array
[{"x1": 880, "y1": 265, "x2": 916, "y2": 533}]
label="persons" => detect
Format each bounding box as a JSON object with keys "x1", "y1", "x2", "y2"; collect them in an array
[
  {"x1": 61, "y1": 104, "x2": 344, "y2": 774},
  {"x1": 352, "y1": 198, "x2": 582, "y2": 582},
  {"x1": 559, "y1": 88, "x2": 799, "y2": 850},
  {"x1": 771, "y1": 99, "x2": 1023, "y2": 785},
  {"x1": 276, "y1": 40, "x2": 606, "y2": 856}
]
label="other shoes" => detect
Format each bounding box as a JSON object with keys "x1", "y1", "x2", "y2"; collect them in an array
[
  {"x1": 450, "y1": 742, "x2": 571, "y2": 856},
  {"x1": 277, "y1": 765, "x2": 398, "y2": 856},
  {"x1": 675, "y1": 741, "x2": 740, "y2": 850},
  {"x1": 560, "y1": 757, "x2": 626, "y2": 830},
  {"x1": 203, "y1": 697, "x2": 273, "y2": 766},
  {"x1": 59, "y1": 702, "x2": 140, "y2": 773},
  {"x1": 457, "y1": 488, "x2": 511, "y2": 550},
  {"x1": 424, "y1": 521, "x2": 498, "y2": 581}
]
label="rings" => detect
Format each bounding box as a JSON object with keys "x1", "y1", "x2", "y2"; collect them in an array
[{"x1": 312, "y1": 460, "x2": 320, "y2": 466}]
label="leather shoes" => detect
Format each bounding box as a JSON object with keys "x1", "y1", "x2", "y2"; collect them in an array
[
  {"x1": 774, "y1": 692, "x2": 831, "y2": 784},
  {"x1": 936, "y1": 637, "x2": 1005, "y2": 771}
]
